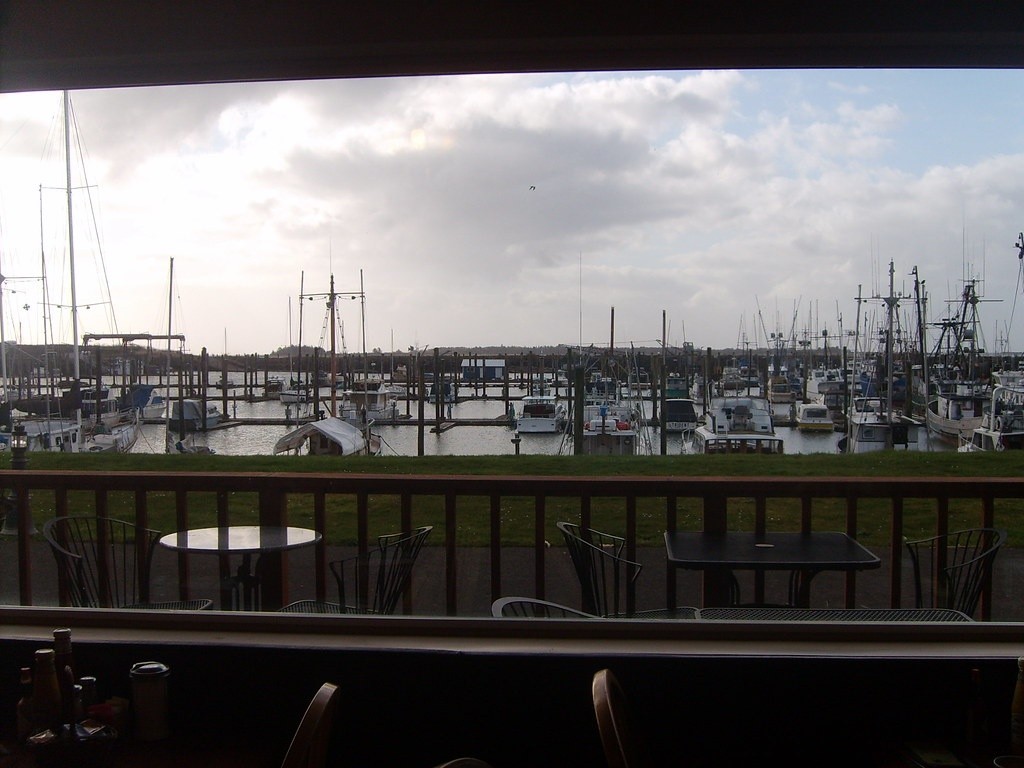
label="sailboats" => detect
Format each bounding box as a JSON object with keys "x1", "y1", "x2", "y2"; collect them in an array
[
  {"x1": 582, "y1": 232, "x2": 1023, "y2": 455},
  {"x1": 0, "y1": 90, "x2": 167, "y2": 456}
]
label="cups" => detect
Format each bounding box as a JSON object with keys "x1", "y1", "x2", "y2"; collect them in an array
[{"x1": 129, "y1": 660, "x2": 170, "y2": 741}]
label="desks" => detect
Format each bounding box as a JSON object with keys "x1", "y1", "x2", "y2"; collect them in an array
[
  {"x1": 662, "y1": 530, "x2": 881, "y2": 608},
  {"x1": 693, "y1": 606, "x2": 972, "y2": 622},
  {"x1": 158, "y1": 526, "x2": 322, "y2": 610}
]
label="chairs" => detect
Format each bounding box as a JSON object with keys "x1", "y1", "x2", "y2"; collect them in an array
[
  {"x1": 490, "y1": 594, "x2": 598, "y2": 621},
  {"x1": 904, "y1": 527, "x2": 1008, "y2": 618},
  {"x1": 591, "y1": 667, "x2": 634, "y2": 768},
  {"x1": 280, "y1": 681, "x2": 341, "y2": 768},
  {"x1": 554, "y1": 520, "x2": 698, "y2": 619},
  {"x1": 43, "y1": 515, "x2": 214, "y2": 610},
  {"x1": 276, "y1": 526, "x2": 433, "y2": 615}
]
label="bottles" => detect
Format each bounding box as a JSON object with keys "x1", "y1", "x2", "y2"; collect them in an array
[
  {"x1": 33, "y1": 649, "x2": 62, "y2": 731},
  {"x1": 45, "y1": 628, "x2": 84, "y2": 736},
  {"x1": 17, "y1": 667, "x2": 36, "y2": 745}
]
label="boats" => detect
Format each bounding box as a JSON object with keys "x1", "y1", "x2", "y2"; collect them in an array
[
  {"x1": 516, "y1": 396, "x2": 567, "y2": 433},
  {"x1": 528, "y1": 377, "x2": 550, "y2": 395},
  {"x1": 273, "y1": 266, "x2": 460, "y2": 458},
  {"x1": 261, "y1": 376, "x2": 288, "y2": 401},
  {"x1": 169, "y1": 398, "x2": 224, "y2": 432}
]
